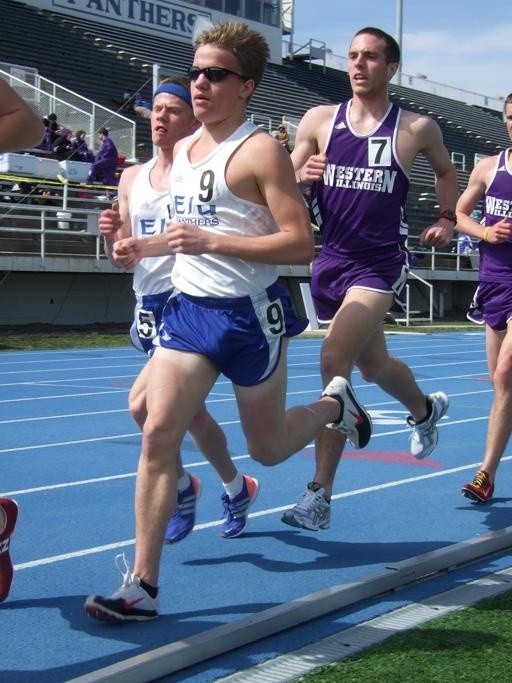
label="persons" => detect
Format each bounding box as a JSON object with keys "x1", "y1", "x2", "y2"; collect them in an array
[
  {"x1": 83, "y1": 18, "x2": 377, "y2": 626},
  {"x1": 96, "y1": 72, "x2": 263, "y2": 549},
  {"x1": 456, "y1": 232, "x2": 480, "y2": 270},
  {"x1": 0, "y1": 75, "x2": 48, "y2": 600},
  {"x1": 274, "y1": 123, "x2": 293, "y2": 154},
  {"x1": 454, "y1": 90, "x2": 512, "y2": 503},
  {"x1": 282, "y1": 25, "x2": 460, "y2": 536},
  {"x1": 23, "y1": 111, "x2": 118, "y2": 206}
]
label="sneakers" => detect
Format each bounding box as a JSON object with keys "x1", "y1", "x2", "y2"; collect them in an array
[
  {"x1": 218, "y1": 475, "x2": 259, "y2": 538},
  {"x1": 321, "y1": 375, "x2": 372, "y2": 448},
  {"x1": 461, "y1": 470, "x2": 495, "y2": 502},
  {"x1": 162, "y1": 471, "x2": 200, "y2": 543},
  {"x1": 282, "y1": 482, "x2": 331, "y2": 531},
  {"x1": 407, "y1": 392, "x2": 448, "y2": 459},
  {"x1": 85, "y1": 553, "x2": 159, "y2": 621},
  {"x1": 0, "y1": 497, "x2": 18, "y2": 602}
]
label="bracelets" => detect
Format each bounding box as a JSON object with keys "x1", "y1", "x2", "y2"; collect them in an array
[
  {"x1": 298, "y1": 168, "x2": 306, "y2": 185},
  {"x1": 483, "y1": 225, "x2": 489, "y2": 240}
]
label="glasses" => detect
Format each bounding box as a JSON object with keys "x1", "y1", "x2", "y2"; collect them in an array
[{"x1": 188, "y1": 66, "x2": 245, "y2": 82}]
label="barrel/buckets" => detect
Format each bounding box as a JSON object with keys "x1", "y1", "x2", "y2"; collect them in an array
[{"x1": 56, "y1": 211, "x2": 72, "y2": 229}]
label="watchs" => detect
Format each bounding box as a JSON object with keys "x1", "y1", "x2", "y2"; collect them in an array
[{"x1": 438, "y1": 208, "x2": 457, "y2": 224}]
